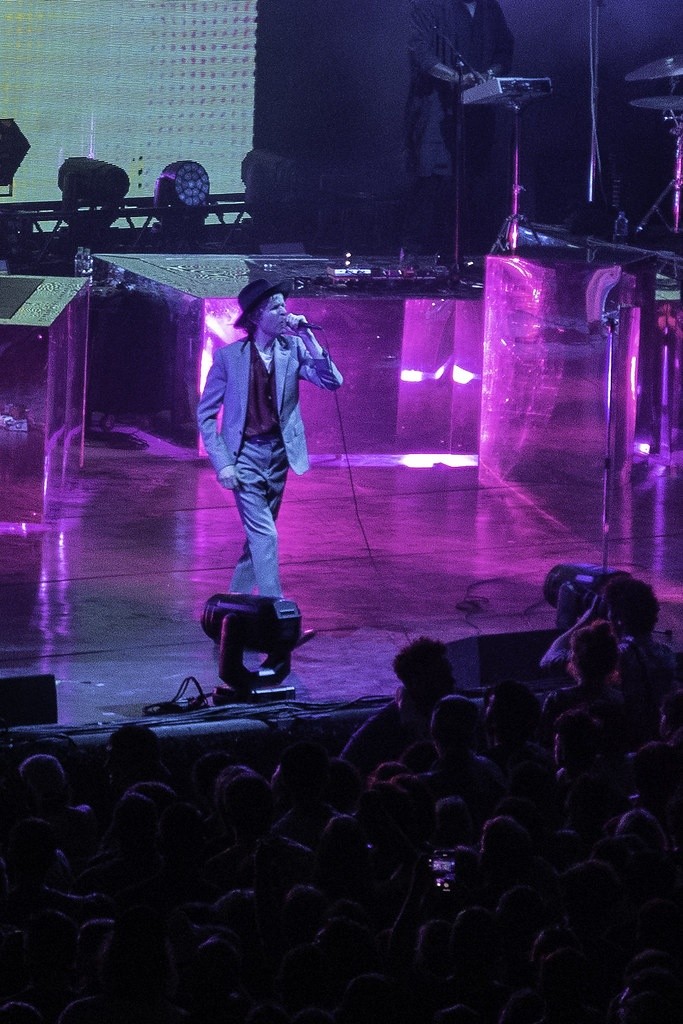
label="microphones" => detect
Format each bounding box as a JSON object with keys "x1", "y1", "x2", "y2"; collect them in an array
[{"x1": 284, "y1": 318, "x2": 320, "y2": 330}]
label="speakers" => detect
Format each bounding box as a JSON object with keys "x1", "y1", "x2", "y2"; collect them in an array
[{"x1": 443, "y1": 627, "x2": 573, "y2": 694}]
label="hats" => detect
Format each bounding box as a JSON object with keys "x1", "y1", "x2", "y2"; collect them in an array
[{"x1": 234, "y1": 276, "x2": 292, "y2": 329}]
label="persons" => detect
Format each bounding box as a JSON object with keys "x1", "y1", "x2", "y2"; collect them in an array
[
  {"x1": 195, "y1": 279, "x2": 346, "y2": 650},
  {"x1": 396, "y1": 0, "x2": 516, "y2": 278},
  {"x1": 0, "y1": 559, "x2": 682, "y2": 1022}
]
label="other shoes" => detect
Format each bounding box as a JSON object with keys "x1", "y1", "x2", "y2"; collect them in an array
[{"x1": 292, "y1": 629, "x2": 316, "y2": 654}]
